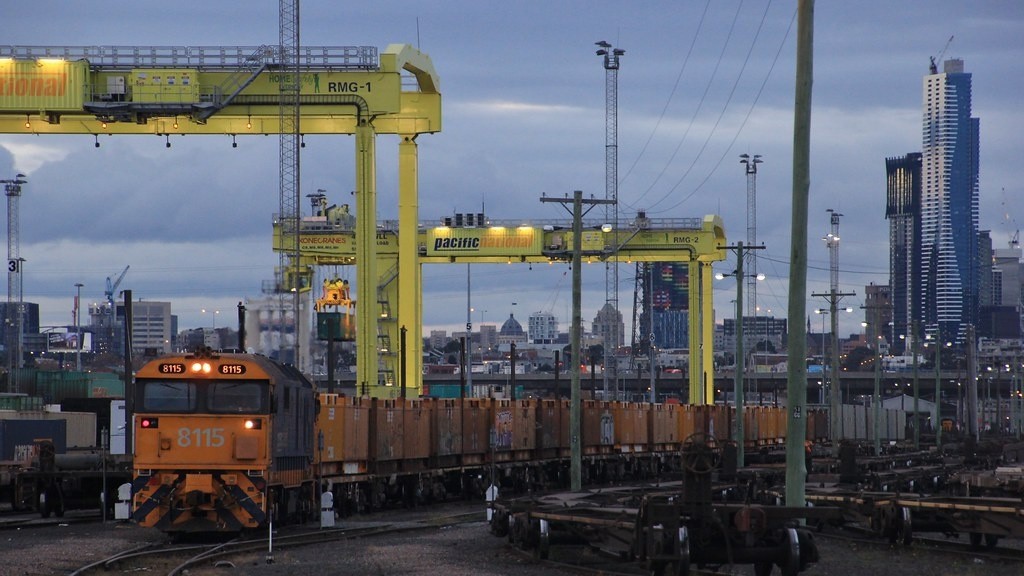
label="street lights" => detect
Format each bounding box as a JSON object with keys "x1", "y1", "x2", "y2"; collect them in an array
[
  {"x1": 740, "y1": 153, "x2": 763, "y2": 406},
  {"x1": 18, "y1": 257, "x2": 26, "y2": 368},
  {"x1": 825, "y1": 208, "x2": 845, "y2": 444},
  {"x1": 74, "y1": 284, "x2": 84, "y2": 372},
  {"x1": 0, "y1": 174, "x2": 27, "y2": 408},
  {"x1": 596, "y1": 41, "x2": 625, "y2": 402}
]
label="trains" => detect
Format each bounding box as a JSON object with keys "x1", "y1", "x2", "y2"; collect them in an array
[{"x1": 132, "y1": 345, "x2": 911, "y2": 539}]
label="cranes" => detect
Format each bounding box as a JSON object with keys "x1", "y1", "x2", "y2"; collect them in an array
[{"x1": 104, "y1": 265, "x2": 129, "y2": 301}]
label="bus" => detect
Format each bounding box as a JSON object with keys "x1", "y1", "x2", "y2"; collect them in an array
[{"x1": 749, "y1": 354, "x2": 788, "y2": 374}]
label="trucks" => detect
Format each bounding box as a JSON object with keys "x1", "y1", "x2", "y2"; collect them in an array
[
  {"x1": 453, "y1": 365, "x2": 486, "y2": 375},
  {"x1": 498, "y1": 366, "x2": 525, "y2": 374}
]
